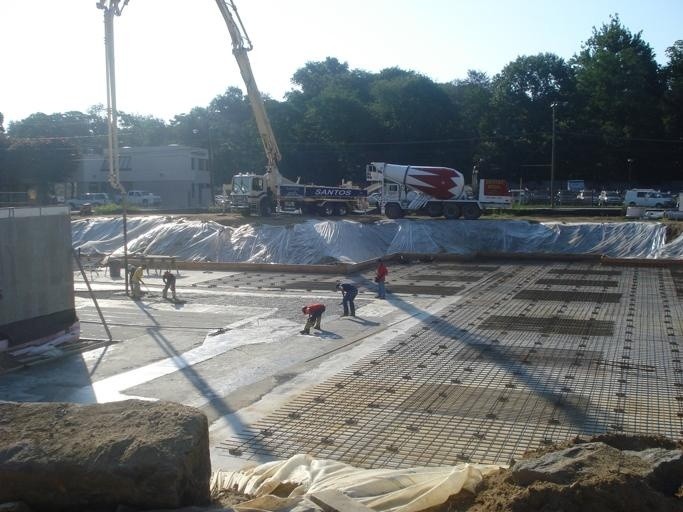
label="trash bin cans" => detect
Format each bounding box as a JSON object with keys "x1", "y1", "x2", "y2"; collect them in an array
[{"x1": 110, "y1": 259, "x2": 121, "y2": 277}]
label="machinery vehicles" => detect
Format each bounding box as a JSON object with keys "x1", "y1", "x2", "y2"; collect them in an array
[{"x1": 93, "y1": 0, "x2": 516, "y2": 221}]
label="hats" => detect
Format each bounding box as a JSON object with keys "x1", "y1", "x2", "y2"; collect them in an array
[
  {"x1": 336, "y1": 284, "x2": 339, "y2": 290},
  {"x1": 377, "y1": 259, "x2": 383, "y2": 262},
  {"x1": 302, "y1": 307, "x2": 307, "y2": 313}
]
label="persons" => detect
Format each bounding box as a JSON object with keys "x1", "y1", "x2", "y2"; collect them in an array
[
  {"x1": 131, "y1": 264, "x2": 147, "y2": 300},
  {"x1": 161, "y1": 271, "x2": 176, "y2": 299},
  {"x1": 336, "y1": 282, "x2": 358, "y2": 316},
  {"x1": 374, "y1": 259, "x2": 388, "y2": 299},
  {"x1": 130, "y1": 264, "x2": 140, "y2": 294},
  {"x1": 300, "y1": 304, "x2": 325, "y2": 334}
]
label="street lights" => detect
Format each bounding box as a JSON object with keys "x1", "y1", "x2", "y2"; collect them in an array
[{"x1": 545, "y1": 100, "x2": 569, "y2": 209}]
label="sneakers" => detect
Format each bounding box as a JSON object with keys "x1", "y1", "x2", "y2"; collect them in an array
[
  {"x1": 300, "y1": 327, "x2": 319, "y2": 334},
  {"x1": 340, "y1": 315, "x2": 355, "y2": 317},
  {"x1": 376, "y1": 296, "x2": 385, "y2": 299}
]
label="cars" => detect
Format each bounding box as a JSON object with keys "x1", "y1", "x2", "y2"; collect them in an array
[
  {"x1": 623, "y1": 187, "x2": 673, "y2": 207},
  {"x1": 508, "y1": 188, "x2": 622, "y2": 206},
  {"x1": 44, "y1": 190, "x2": 161, "y2": 207}
]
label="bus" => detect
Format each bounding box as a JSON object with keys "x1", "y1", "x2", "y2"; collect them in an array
[{"x1": 0, "y1": 192, "x2": 27, "y2": 207}]
